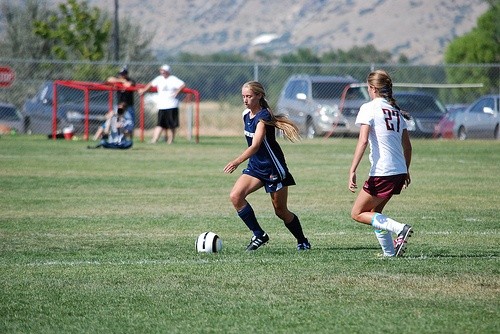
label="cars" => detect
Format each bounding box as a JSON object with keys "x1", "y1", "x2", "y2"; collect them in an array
[
  {"x1": 0, "y1": 102, "x2": 29, "y2": 134},
  {"x1": 24, "y1": 80, "x2": 120, "y2": 138},
  {"x1": 455, "y1": 94, "x2": 500, "y2": 140},
  {"x1": 442, "y1": 101, "x2": 472, "y2": 129}
]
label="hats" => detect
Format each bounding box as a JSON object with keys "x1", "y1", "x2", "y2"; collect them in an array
[{"x1": 158, "y1": 63, "x2": 171, "y2": 74}]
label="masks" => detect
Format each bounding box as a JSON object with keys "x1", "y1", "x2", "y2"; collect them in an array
[{"x1": 118, "y1": 108, "x2": 124, "y2": 115}]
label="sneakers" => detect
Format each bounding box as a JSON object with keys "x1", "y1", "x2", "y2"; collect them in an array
[
  {"x1": 245, "y1": 232, "x2": 269, "y2": 251},
  {"x1": 393, "y1": 224, "x2": 413, "y2": 258},
  {"x1": 296, "y1": 240, "x2": 311, "y2": 252}
]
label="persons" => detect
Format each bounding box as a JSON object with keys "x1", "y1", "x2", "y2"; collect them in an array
[
  {"x1": 139, "y1": 64, "x2": 186, "y2": 147},
  {"x1": 349, "y1": 70, "x2": 414, "y2": 260},
  {"x1": 103, "y1": 103, "x2": 135, "y2": 147},
  {"x1": 94, "y1": 67, "x2": 137, "y2": 142},
  {"x1": 222, "y1": 81, "x2": 313, "y2": 253}
]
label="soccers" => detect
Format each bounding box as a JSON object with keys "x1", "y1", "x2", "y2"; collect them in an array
[{"x1": 194, "y1": 232, "x2": 222, "y2": 254}]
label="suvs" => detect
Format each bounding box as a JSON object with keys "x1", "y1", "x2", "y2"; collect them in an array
[
  {"x1": 275, "y1": 73, "x2": 379, "y2": 139},
  {"x1": 387, "y1": 90, "x2": 451, "y2": 137}
]
